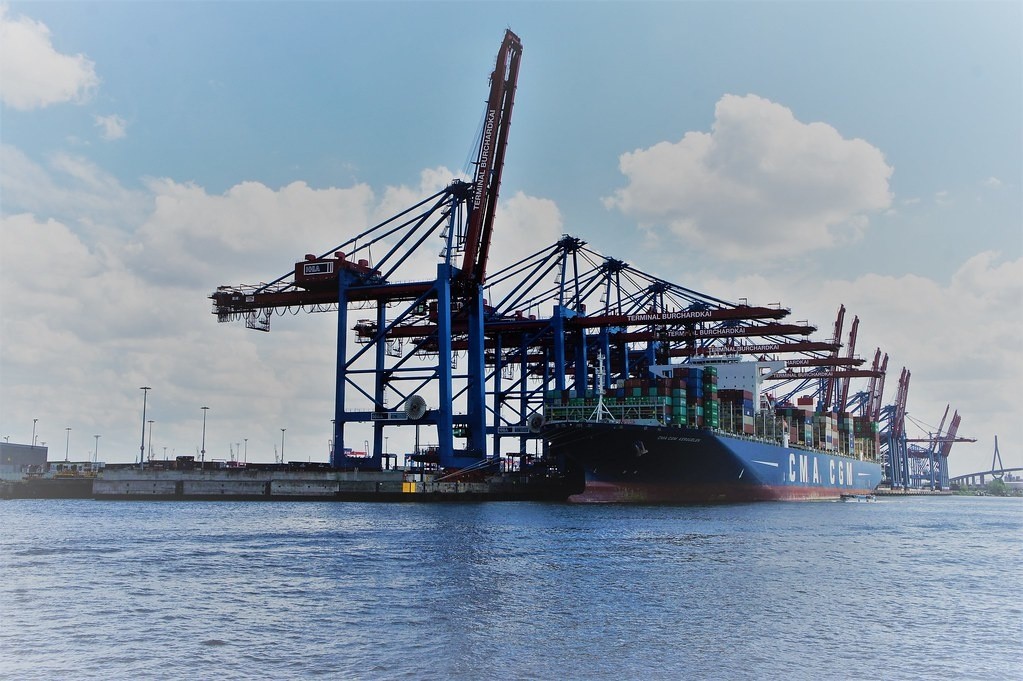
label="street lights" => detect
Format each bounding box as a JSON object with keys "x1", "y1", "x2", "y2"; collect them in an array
[
  {"x1": 281, "y1": 429, "x2": 286, "y2": 464},
  {"x1": 94, "y1": 435, "x2": 101, "y2": 462},
  {"x1": 201, "y1": 407, "x2": 210, "y2": 468},
  {"x1": 384, "y1": 437, "x2": 388, "y2": 453},
  {"x1": 32, "y1": 419, "x2": 38, "y2": 446},
  {"x1": 140, "y1": 387, "x2": 151, "y2": 469},
  {"x1": 244, "y1": 439, "x2": 248, "y2": 464},
  {"x1": 65, "y1": 428, "x2": 71, "y2": 463},
  {"x1": 163, "y1": 447, "x2": 167, "y2": 460},
  {"x1": 148, "y1": 420, "x2": 155, "y2": 460},
  {"x1": 4, "y1": 436, "x2": 10, "y2": 443},
  {"x1": 236, "y1": 443, "x2": 240, "y2": 468}
]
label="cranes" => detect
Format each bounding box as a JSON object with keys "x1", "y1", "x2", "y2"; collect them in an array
[
  {"x1": 207, "y1": 27, "x2": 524, "y2": 482},
  {"x1": 350, "y1": 233, "x2": 977, "y2": 491}
]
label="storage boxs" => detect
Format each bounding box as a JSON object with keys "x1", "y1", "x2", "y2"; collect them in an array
[{"x1": 547, "y1": 366, "x2": 882, "y2": 463}]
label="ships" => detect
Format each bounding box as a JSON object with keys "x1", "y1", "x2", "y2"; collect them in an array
[{"x1": 542, "y1": 347, "x2": 883, "y2": 503}]
